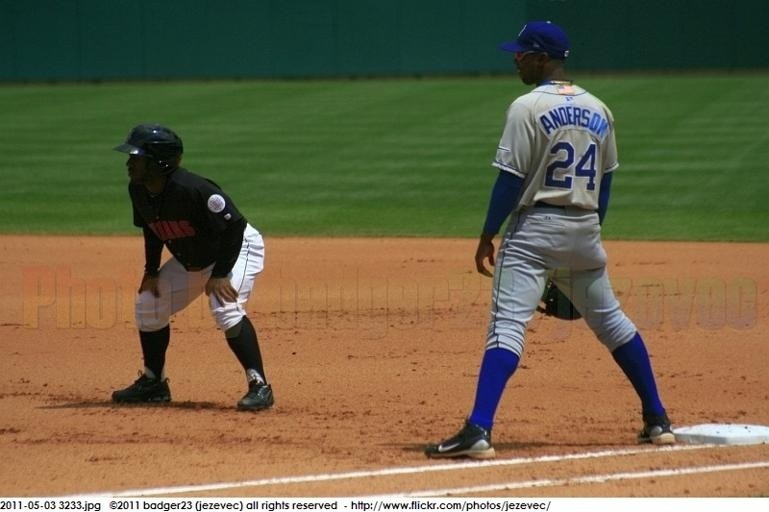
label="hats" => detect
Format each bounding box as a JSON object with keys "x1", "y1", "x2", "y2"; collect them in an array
[
  {"x1": 497, "y1": 20, "x2": 571, "y2": 62},
  {"x1": 111, "y1": 123, "x2": 185, "y2": 164}
]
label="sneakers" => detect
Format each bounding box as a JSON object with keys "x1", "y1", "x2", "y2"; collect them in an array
[
  {"x1": 423, "y1": 421, "x2": 497, "y2": 459},
  {"x1": 237, "y1": 383, "x2": 276, "y2": 411},
  {"x1": 636, "y1": 423, "x2": 676, "y2": 447},
  {"x1": 111, "y1": 367, "x2": 176, "y2": 406}
]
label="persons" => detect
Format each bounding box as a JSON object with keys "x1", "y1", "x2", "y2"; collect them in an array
[
  {"x1": 112, "y1": 123, "x2": 275, "y2": 412},
  {"x1": 426, "y1": 17, "x2": 679, "y2": 461}
]
